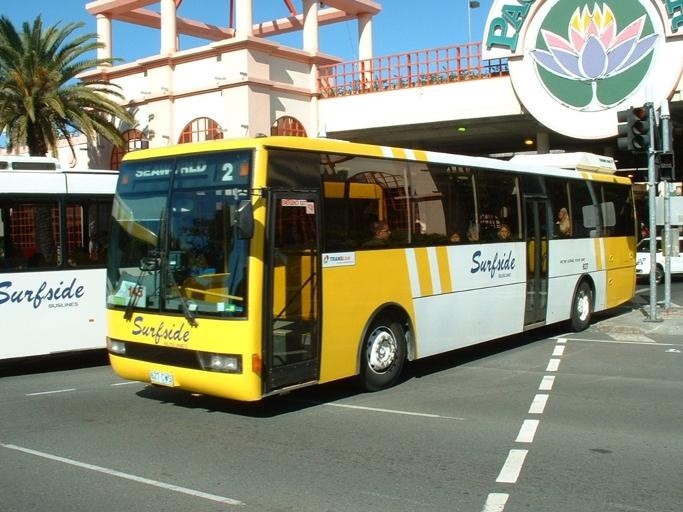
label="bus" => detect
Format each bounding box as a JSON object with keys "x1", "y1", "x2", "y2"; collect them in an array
[
  {"x1": 0, "y1": 154, "x2": 121, "y2": 362},
  {"x1": 104, "y1": 135, "x2": 643, "y2": 403}
]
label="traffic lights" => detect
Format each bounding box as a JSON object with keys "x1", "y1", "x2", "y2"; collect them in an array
[
  {"x1": 631, "y1": 105, "x2": 651, "y2": 151},
  {"x1": 614, "y1": 107, "x2": 635, "y2": 153},
  {"x1": 657, "y1": 150, "x2": 676, "y2": 182}
]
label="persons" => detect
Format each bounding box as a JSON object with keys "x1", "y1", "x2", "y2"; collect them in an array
[
  {"x1": 362, "y1": 221, "x2": 391, "y2": 247},
  {"x1": 447, "y1": 232, "x2": 462, "y2": 244},
  {"x1": 495, "y1": 224, "x2": 512, "y2": 241},
  {"x1": 555, "y1": 207, "x2": 569, "y2": 236}
]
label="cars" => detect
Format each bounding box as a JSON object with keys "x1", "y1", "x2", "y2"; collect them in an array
[{"x1": 635, "y1": 234, "x2": 683, "y2": 285}]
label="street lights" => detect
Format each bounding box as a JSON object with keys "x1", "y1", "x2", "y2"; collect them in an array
[{"x1": 468, "y1": 0, "x2": 480, "y2": 74}]
